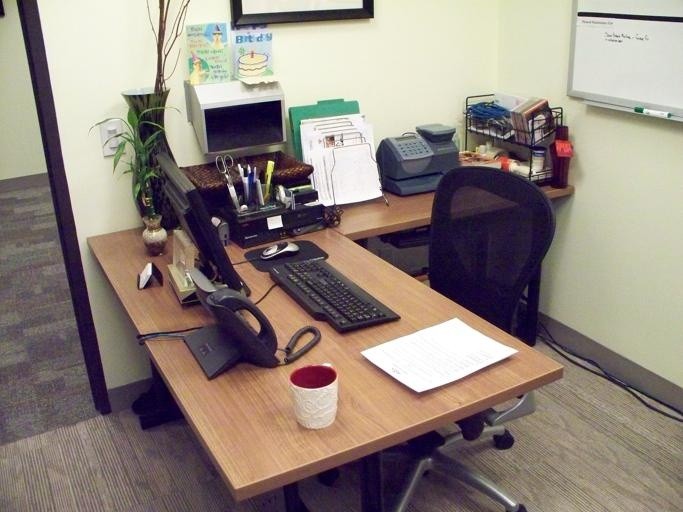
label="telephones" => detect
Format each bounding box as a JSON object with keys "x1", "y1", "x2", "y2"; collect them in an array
[{"x1": 184, "y1": 289, "x2": 280, "y2": 381}]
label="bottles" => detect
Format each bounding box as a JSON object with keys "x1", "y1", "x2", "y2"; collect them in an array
[{"x1": 141, "y1": 214, "x2": 166, "y2": 255}]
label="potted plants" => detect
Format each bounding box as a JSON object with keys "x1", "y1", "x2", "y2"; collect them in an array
[{"x1": 87, "y1": 104, "x2": 183, "y2": 257}]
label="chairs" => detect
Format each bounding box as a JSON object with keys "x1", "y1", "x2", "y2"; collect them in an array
[{"x1": 314, "y1": 166, "x2": 555, "y2": 512}]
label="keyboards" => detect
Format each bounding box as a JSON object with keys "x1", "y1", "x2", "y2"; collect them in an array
[{"x1": 269, "y1": 260, "x2": 401, "y2": 333}]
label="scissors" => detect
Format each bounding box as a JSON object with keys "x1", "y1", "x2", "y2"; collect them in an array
[{"x1": 215, "y1": 154, "x2": 234, "y2": 185}]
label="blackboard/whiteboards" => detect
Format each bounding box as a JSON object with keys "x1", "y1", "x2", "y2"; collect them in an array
[{"x1": 566, "y1": 0, "x2": 683, "y2": 122}]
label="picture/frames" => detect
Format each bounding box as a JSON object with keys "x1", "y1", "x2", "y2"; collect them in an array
[{"x1": 228, "y1": 0, "x2": 375, "y2": 29}]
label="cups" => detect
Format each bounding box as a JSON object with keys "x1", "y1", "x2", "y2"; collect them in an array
[{"x1": 288, "y1": 363, "x2": 339, "y2": 430}]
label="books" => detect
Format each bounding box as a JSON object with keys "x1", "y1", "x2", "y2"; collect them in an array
[{"x1": 510, "y1": 97, "x2": 556, "y2": 146}]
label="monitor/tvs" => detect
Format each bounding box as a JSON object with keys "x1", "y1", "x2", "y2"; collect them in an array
[{"x1": 155, "y1": 151, "x2": 251, "y2": 305}]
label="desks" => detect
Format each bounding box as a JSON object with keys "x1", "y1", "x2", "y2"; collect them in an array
[{"x1": 85, "y1": 184, "x2": 574, "y2": 512}]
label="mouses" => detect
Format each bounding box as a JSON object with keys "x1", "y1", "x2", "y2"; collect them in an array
[{"x1": 260, "y1": 242, "x2": 300, "y2": 261}]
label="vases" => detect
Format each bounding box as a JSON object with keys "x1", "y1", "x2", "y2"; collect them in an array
[{"x1": 120, "y1": 85, "x2": 190, "y2": 232}]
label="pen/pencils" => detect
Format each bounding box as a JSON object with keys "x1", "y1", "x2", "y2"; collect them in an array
[{"x1": 237, "y1": 161, "x2": 275, "y2": 205}]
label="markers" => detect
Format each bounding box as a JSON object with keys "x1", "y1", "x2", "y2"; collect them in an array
[{"x1": 634, "y1": 107, "x2": 672, "y2": 118}]
